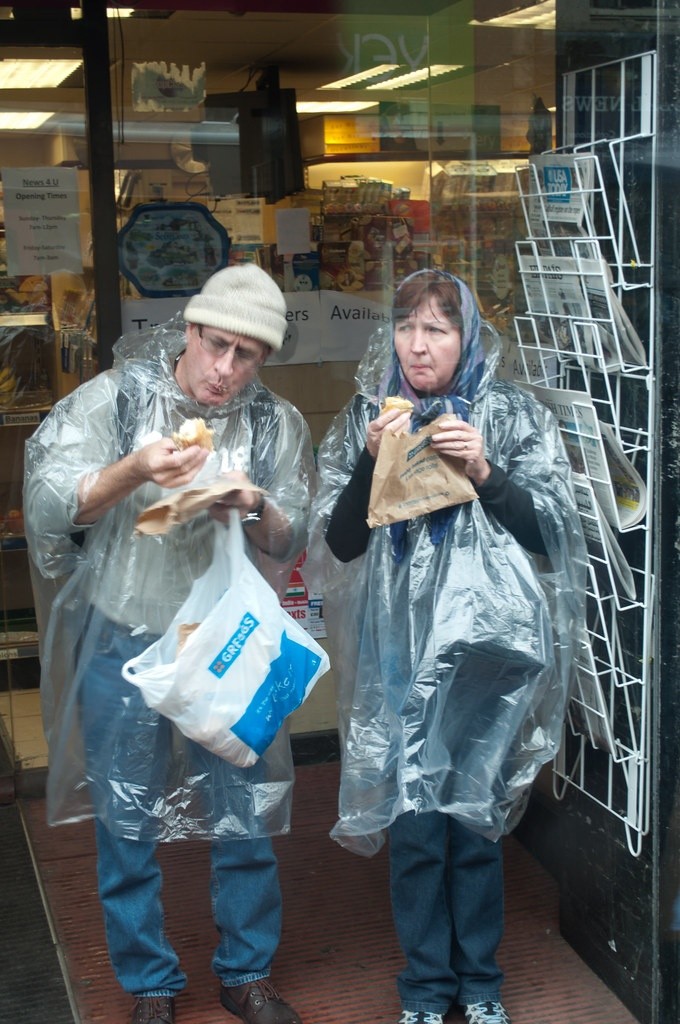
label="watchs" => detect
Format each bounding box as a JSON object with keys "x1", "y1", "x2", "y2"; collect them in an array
[{"x1": 241, "y1": 491, "x2": 266, "y2": 527}]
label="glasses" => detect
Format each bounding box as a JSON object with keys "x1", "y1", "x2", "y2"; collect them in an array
[{"x1": 196, "y1": 324, "x2": 266, "y2": 370}]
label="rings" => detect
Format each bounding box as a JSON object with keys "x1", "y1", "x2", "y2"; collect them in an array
[{"x1": 464, "y1": 441, "x2": 469, "y2": 451}]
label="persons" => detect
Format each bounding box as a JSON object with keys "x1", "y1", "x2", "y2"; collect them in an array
[
  {"x1": 553, "y1": 224, "x2": 573, "y2": 256},
  {"x1": 318, "y1": 268, "x2": 584, "y2": 1024},
  {"x1": 23, "y1": 261, "x2": 317, "y2": 1024},
  {"x1": 526, "y1": 97, "x2": 553, "y2": 155},
  {"x1": 385, "y1": 104, "x2": 416, "y2": 151}
]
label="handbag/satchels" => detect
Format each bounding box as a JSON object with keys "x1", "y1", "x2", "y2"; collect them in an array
[{"x1": 122, "y1": 511, "x2": 330, "y2": 770}]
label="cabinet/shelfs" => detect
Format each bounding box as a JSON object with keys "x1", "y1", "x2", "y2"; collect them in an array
[{"x1": 431, "y1": 51, "x2": 660, "y2": 857}]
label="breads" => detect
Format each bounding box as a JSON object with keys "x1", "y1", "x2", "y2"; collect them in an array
[
  {"x1": 380, "y1": 396, "x2": 415, "y2": 414},
  {"x1": 171, "y1": 417, "x2": 214, "y2": 453}
]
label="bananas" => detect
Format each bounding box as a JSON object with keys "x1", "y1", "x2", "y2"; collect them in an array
[{"x1": 0, "y1": 366, "x2": 17, "y2": 403}]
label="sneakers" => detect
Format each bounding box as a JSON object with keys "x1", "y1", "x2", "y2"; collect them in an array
[
  {"x1": 397, "y1": 1007, "x2": 445, "y2": 1024},
  {"x1": 462, "y1": 1002, "x2": 513, "y2": 1024},
  {"x1": 220, "y1": 975, "x2": 303, "y2": 1024},
  {"x1": 129, "y1": 992, "x2": 175, "y2": 1023}
]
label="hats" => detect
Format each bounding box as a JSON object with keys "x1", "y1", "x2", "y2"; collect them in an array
[{"x1": 183, "y1": 262, "x2": 287, "y2": 352}]
